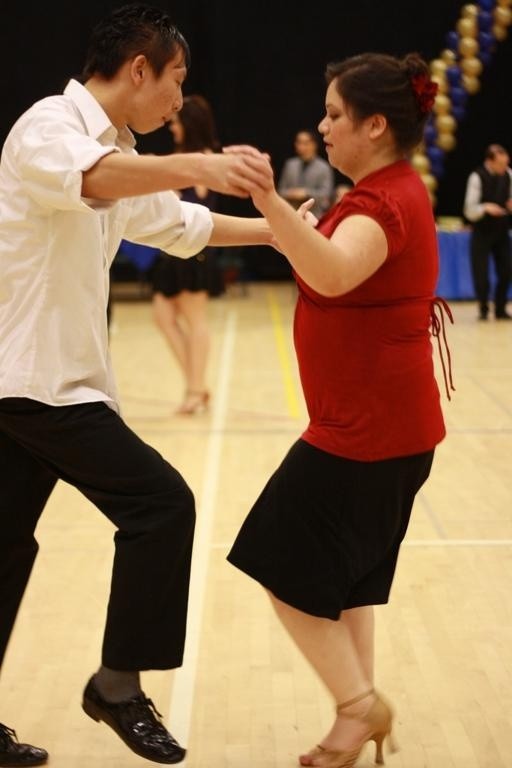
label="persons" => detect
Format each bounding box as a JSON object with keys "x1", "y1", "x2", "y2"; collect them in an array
[
  {"x1": 277, "y1": 128, "x2": 335, "y2": 219},
  {"x1": 148, "y1": 94, "x2": 229, "y2": 418},
  {"x1": 221, "y1": 50, "x2": 448, "y2": 768},
  {"x1": 462, "y1": 142, "x2": 512, "y2": 321},
  {"x1": 0, "y1": 3, "x2": 322, "y2": 768}
]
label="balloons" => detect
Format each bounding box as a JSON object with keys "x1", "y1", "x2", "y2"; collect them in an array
[{"x1": 405, "y1": 0, "x2": 512, "y2": 208}]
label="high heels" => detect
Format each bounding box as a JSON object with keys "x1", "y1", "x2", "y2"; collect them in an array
[
  {"x1": 173, "y1": 387, "x2": 210, "y2": 415},
  {"x1": 298, "y1": 690, "x2": 392, "y2": 768}
]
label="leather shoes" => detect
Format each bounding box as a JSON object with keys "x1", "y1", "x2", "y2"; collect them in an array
[
  {"x1": 80, "y1": 674, "x2": 187, "y2": 765},
  {"x1": 0, "y1": 721, "x2": 49, "y2": 767}
]
